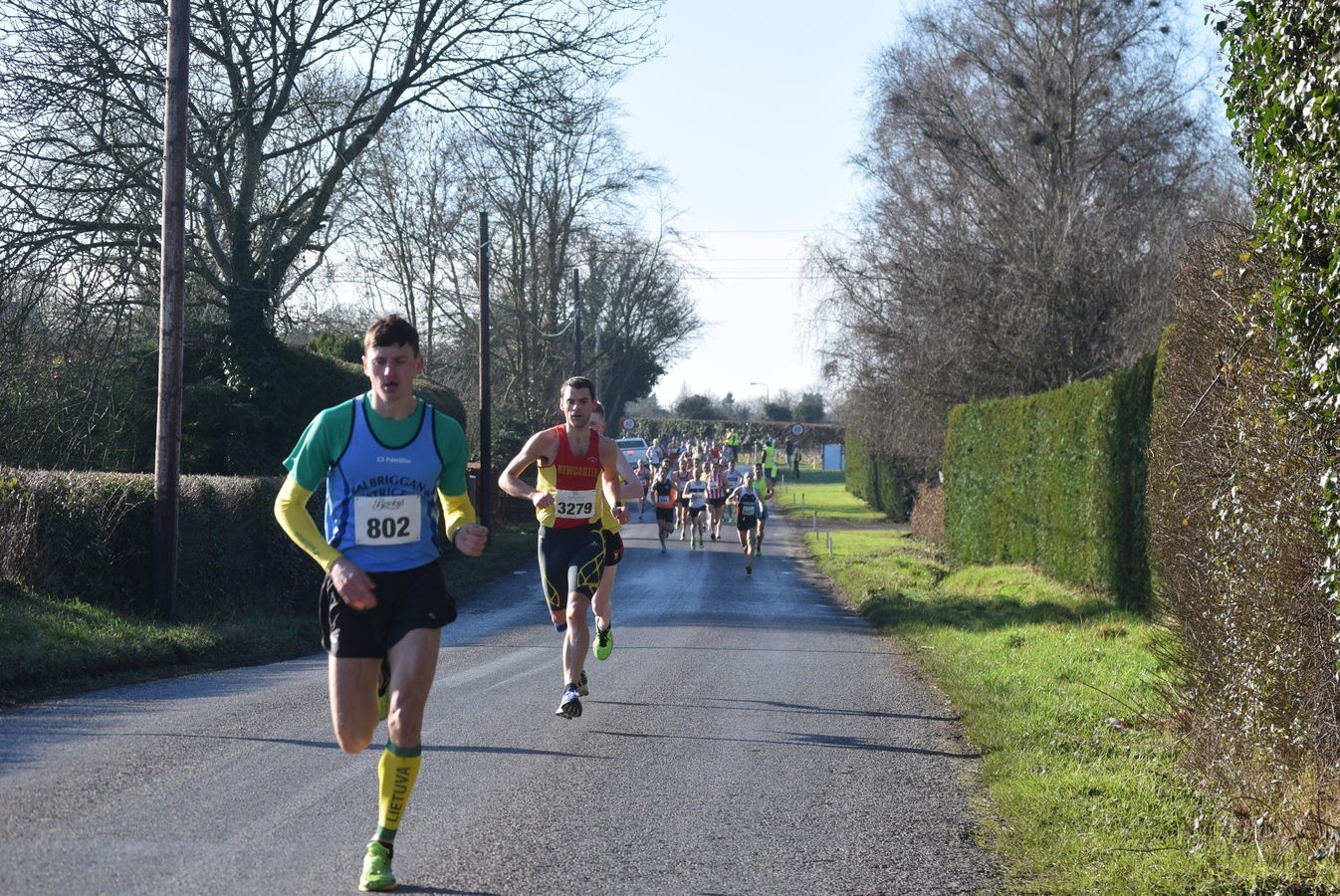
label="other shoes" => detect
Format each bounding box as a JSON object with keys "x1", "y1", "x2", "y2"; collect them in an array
[
  {"x1": 674, "y1": 506, "x2": 736, "y2": 550},
  {"x1": 756, "y1": 547, "x2": 762, "y2": 555},
  {"x1": 661, "y1": 546, "x2": 666, "y2": 553},
  {"x1": 746, "y1": 565, "x2": 752, "y2": 574}
]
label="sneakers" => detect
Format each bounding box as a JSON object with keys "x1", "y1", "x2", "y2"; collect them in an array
[
  {"x1": 555, "y1": 687, "x2": 582, "y2": 720},
  {"x1": 376, "y1": 658, "x2": 391, "y2": 721},
  {"x1": 554, "y1": 621, "x2": 567, "y2": 632},
  {"x1": 359, "y1": 841, "x2": 397, "y2": 892},
  {"x1": 577, "y1": 669, "x2": 589, "y2": 696},
  {"x1": 593, "y1": 616, "x2": 613, "y2": 660}
]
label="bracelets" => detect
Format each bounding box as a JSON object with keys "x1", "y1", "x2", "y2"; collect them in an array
[
  {"x1": 451, "y1": 526, "x2": 463, "y2": 554},
  {"x1": 609, "y1": 501, "x2": 621, "y2": 508}
]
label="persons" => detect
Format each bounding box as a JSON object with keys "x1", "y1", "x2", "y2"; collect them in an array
[
  {"x1": 498, "y1": 376, "x2": 630, "y2": 720},
  {"x1": 682, "y1": 467, "x2": 710, "y2": 550},
  {"x1": 633, "y1": 427, "x2": 800, "y2": 542},
  {"x1": 578, "y1": 399, "x2": 644, "y2": 696},
  {"x1": 273, "y1": 314, "x2": 489, "y2": 892},
  {"x1": 725, "y1": 471, "x2": 765, "y2": 575},
  {"x1": 650, "y1": 465, "x2": 681, "y2": 554}
]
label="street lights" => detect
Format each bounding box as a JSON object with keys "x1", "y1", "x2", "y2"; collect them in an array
[{"x1": 750, "y1": 383, "x2": 769, "y2": 405}]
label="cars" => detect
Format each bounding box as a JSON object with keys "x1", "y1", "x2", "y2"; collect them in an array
[{"x1": 616, "y1": 438, "x2": 650, "y2": 467}]
